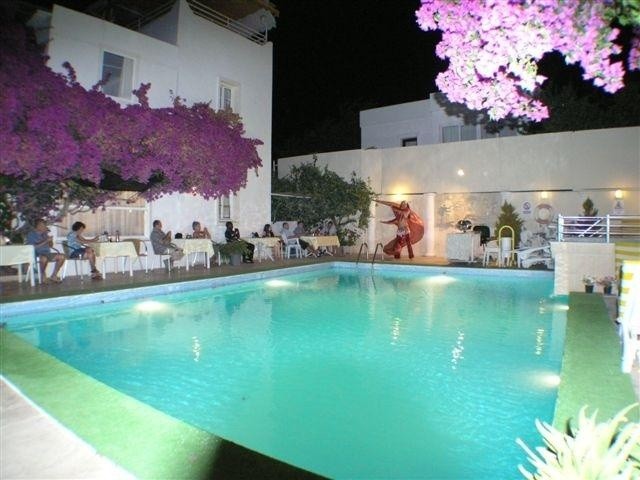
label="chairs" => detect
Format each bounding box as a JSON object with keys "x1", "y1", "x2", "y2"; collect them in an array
[
  {"x1": 498, "y1": 225, "x2": 516, "y2": 269},
  {"x1": 25, "y1": 231, "x2": 302, "y2": 287}
]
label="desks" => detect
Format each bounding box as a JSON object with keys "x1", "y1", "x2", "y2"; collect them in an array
[
  {"x1": 446, "y1": 232, "x2": 480, "y2": 264},
  {"x1": 300, "y1": 235, "x2": 341, "y2": 259},
  {"x1": 0, "y1": 243, "x2": 37, "y2": 288},
  {"x1": 483, "y1": 247, "x2": 521, "y2": 268}
]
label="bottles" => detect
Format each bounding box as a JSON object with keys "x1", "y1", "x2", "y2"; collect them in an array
[
  {"x1": 116, "y1": 231, "x2": 119, "y2": 241},
  {"x1": 99, "y1": 231, "x2": 111, "y2": 241}
]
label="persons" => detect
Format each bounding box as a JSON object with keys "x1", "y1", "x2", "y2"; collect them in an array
[
  {"x1": 282, "y1": 223, "x2": 322, "y2": 257},
  {"x1": 225, "y1": 222, "x2": 254, "y2": 263},
  {"x1": 27, "y1": 218, "x2": 65, "y2": 285},
  {"x1": 150, "y1": 221, "x2": 184, "y2": 272},
  {"x1": 67, "y1": 222, "x2": 104, "y2": 280},
  {"x1": 294, "y1": 221, "x2": 308, "y2": 236},
  {"x1": 262, "y1": 224, "x2": 274, "y2": 237},
  {"x1": 317, "y1": 222, "x2": 336, "y2": 235},
  {"x1": 192, "y1": 222, "x2": 218, "y2": 267},
  {"x1": 388, "y1": 201, "x2": 414, "y2": 260}
]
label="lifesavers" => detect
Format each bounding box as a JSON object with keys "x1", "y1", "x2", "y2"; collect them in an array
[{"x1": 534, "y1": 205, "x2": 553, "y2": 225}]
